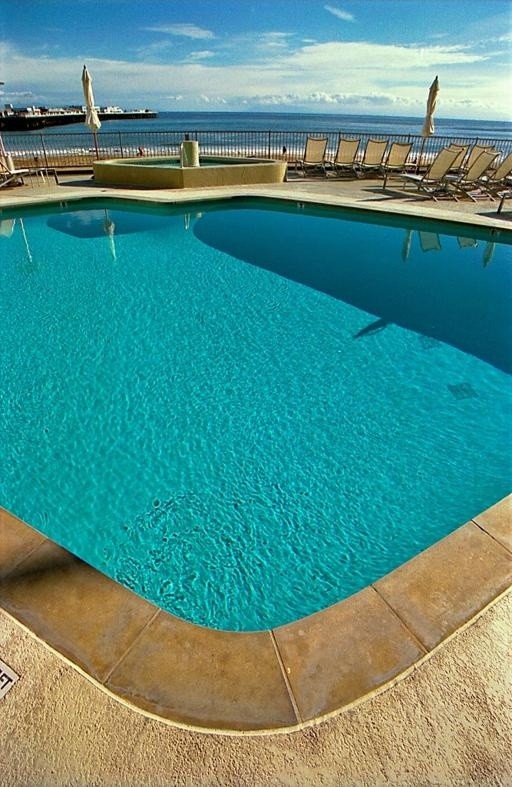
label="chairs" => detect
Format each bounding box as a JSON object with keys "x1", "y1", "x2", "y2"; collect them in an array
[
  {"x1": 400, "y1": 228, "x2": 499, "y2": 265},
  {"x1": 0, "y1": 159, "x2": 59, "y2": 188},
  {"x1": 296, "y1": 136, "x2": 512, "y2": 203}
]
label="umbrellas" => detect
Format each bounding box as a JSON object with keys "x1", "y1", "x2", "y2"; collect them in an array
[
  {"x1": 81, "y1": 65, "x2": 110, "y2": 160},
  {"x1": 414, "y1": 75, "x2": 440, "y2": 175}
]
